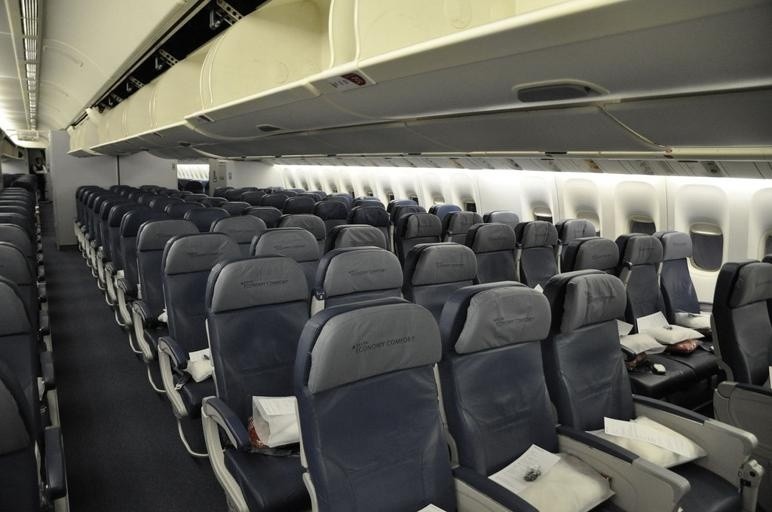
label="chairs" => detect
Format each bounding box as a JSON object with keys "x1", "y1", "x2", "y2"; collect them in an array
[{"x1": 0, "y1": 170, "x2": 69, "y2": 511}]
